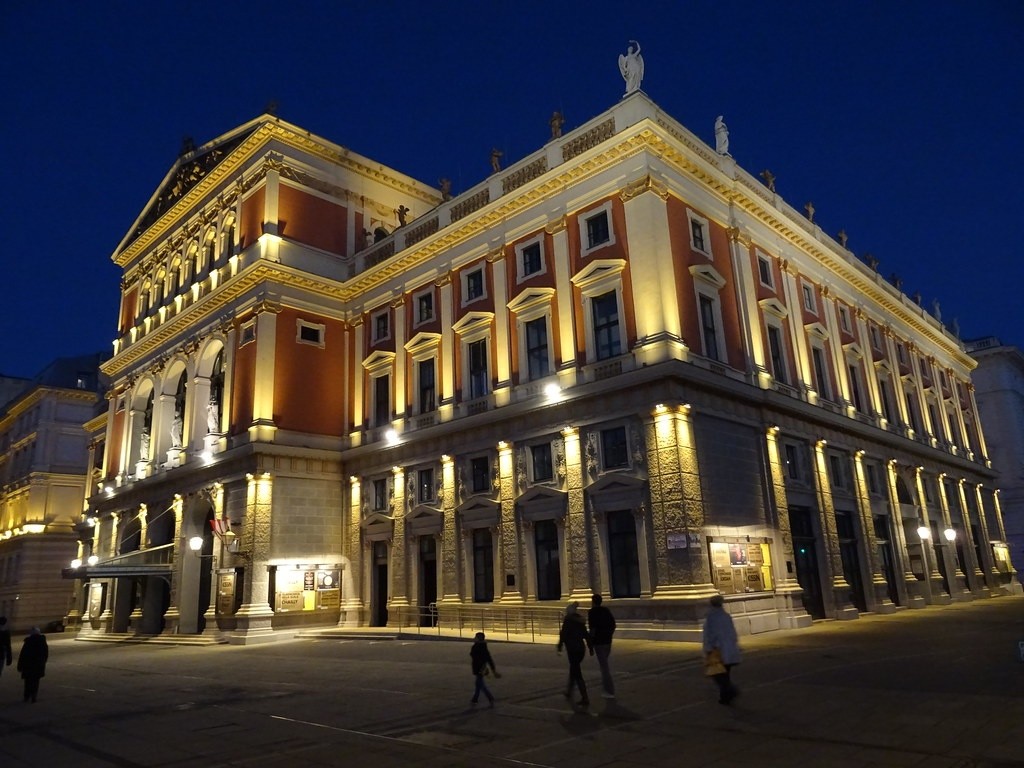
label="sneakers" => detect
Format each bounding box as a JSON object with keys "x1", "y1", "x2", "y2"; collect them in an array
[{"x1": 601, "y1": 692, "x2": 616, "y2": 699}]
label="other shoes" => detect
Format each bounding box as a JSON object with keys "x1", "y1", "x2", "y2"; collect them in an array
[
  {"x1": 561, "y1": 690, "x2": 571, "y2": 699},
  {"x1": 488, "y1": 698, "x2": 495, "y2": 709},
  {"x1": 469, "y1": 699, "x2": 478, "y2": 705},
  {"x1": 576, "y1": 700, "x2": 589, "y2": 706}
]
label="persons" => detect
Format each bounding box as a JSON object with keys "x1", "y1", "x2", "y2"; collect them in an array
[
  {"x1": 715, "y1": 115, "x2": 730, "y2": 154},
  {"x1": 557, "y1": 601, "x2": 595, "y2": 705},
  {"x1": 0, "y1": 617, "x2": 12, "y2": 677},
  {"x1": 624, "y1": 40, "x2": 641, "y2": 92},
  {"x1": 469, "y1": 632, "x2": 501, "y2": 709},
  {"x1": 588, "y1": 594, "x2": 617, "y2": 699},
  {"x1": 703, "y1": 595, "x2": 740, "y2": 704},
  {"x1": 16, "y1": 627, "x2": 49, "y2": 703}
]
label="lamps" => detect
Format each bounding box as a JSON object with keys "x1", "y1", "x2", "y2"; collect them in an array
[
  {"x1": 190, "y1": 537, "x2": 217, "y2": 564},
  {"x1": 220, "y1": 526, "x2": 248, "y2": 558}
]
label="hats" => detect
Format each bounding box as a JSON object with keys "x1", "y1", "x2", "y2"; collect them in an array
[
  {"x1": 565, "y1": 605, "x2": 577, "y2": 614},
  {"x1": 476, "y1": 633, "x2": 485, "y2": 641},
  {"x1": 31, "y1": 626, "x2": 41, "y2": 636}
]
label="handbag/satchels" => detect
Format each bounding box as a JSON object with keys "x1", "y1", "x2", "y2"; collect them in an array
[{"x1": 703, "y1": 648, "x2": 726, "y2": 677}]
